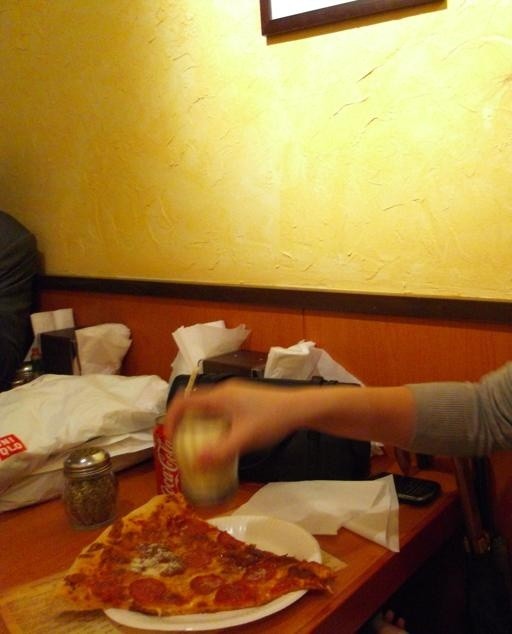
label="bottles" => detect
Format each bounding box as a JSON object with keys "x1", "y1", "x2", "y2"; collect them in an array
[{"x1": 63, "y1": 446, "x2": 118, "y2": 532}]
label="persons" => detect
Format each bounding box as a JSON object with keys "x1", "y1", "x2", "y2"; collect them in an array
[
  {"x1": 160, "y1": 357, "x2": 511, "y2": 470},
  {"x1": 0, "y1": 206, "x2": 44, "y2": 398}
]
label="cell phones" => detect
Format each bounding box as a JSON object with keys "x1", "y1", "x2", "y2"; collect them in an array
[{"x1": 367, "y1": 472, "x2": 441, "y2": 504}]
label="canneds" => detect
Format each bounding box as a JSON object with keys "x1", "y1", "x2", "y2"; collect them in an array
[{"x1": 153, "y1": 415, "x2": 190, "y2": 496}]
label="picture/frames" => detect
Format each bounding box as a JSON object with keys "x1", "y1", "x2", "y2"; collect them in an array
[{"x1": 260, "y1": 0, "x2": 446, "y2": 36}]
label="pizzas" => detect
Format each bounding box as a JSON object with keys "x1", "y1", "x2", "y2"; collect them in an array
[{"x1": 54, "y1": 492, "x2": 340, "y2": 616}]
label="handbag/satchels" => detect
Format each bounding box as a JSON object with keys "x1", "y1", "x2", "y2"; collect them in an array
[{"x1": 162, "y1": 368, "x2": 372, "y2": 483}]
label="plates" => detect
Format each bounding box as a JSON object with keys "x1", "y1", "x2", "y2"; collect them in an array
[{"x1": 98, "y1": 514, "x2": 323, "y2": 632}]
